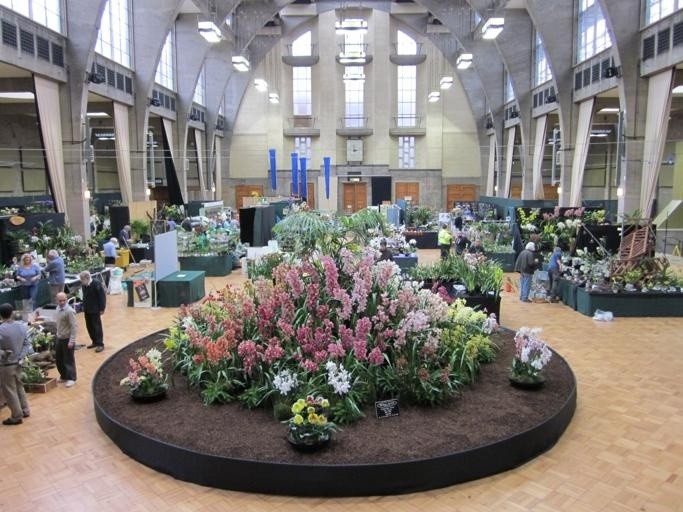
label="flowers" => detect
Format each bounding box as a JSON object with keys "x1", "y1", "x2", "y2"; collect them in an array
[
  {"x1": 512, "y1": 326, "x2": 552, "y2": 376},
  {"x1": 283, "y1": 395, "x2": 341, "y2": 440},
  {"x1": 119, "y1": 348, "x2": 169, "y2": 394}
]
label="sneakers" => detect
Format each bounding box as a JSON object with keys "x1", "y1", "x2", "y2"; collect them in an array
[
  {"x1": 94, "y1": 345, "x2": 104, "y2": 351},
  {"x1": 55, "y1": 375, "x2": 66, "y2": 383},
  {"x1": 523, "y1": 299, "x2": 532, "y2": 303},
  {"x1": 64, "y1": 378, "x2": 76, "y2": 387},
  {"x1": 2, "y1": 418, "x2": 22, "y2": 425},
  {"x1": 20, "y1": 413, "x2": 29, "y2": 417},
  {"x1": 86, "y1": 341, "x2": 96, "y2": 349}
]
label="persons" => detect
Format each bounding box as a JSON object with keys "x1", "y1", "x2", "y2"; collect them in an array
[
  {"x1": 54, "y1": 292, "x2": 76, "y2": 388},
  {"x1": 547, "y1": 246, "x2": 563, "y2": 303},
  {"x1": 79, "y1": 270, "x2": 106, "y2": 353},
  {"x1": 44, "y1": 249, "x2": 65, "y2": 304},
  {"x1": 16, "y1": 253, "x2": 42, "y2": 311},
  {"x1": 166, "y1": 218, "x2": 177, "y2": 231},
  {"x1": 0, "y1": 302, "x2": 30, "y2": 425},
  {"x1": 224, "y1": 218, "x2": 231, "y2": 228},
  {"x1": 104, "y1": 237, "x2": 118, "y2": 267},
  {"x1": 377, "y1": 239, "x2": 394, "y2": 261},
  {"x1": 437, "y1": 212, "x2": 487, "y2": 260},
  {"x1": 119, "y1": 224, "x2": 131, "y2": 249},
  {"x1": 513, "y1": 242, "x2": 540, "y2": 302},
  {"x1": 230, "y1": 216, "x2": 240, "y2": 229}
]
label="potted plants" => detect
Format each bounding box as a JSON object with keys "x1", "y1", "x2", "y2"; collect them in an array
[
  {"x1": 24, "y1": 366, "x2": 57, "y2": 392},
  {"x1": 31, "y1": 333, "x2": 52, "y2": 352}
]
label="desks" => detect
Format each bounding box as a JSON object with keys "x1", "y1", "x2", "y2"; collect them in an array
[{"x1": 390, "y1": 220, "x2": 683, "y2": 319}]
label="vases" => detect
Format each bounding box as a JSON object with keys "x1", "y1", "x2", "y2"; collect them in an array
[
  {"x1": 131, "y1": 389, "x2": 165, "y2": 403},
  {"x1": 509, "y1": 371, "x2": 546, "y2": 389},
  {"x1": 286, "y1": 428, "x2": 332, "y2": 453}
]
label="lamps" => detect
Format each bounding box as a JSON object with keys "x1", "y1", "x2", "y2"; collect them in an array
[
  {"x1": 198, "y1": 1, "x2": 280, "y2": 104},
  {"x1": 429, "y1": 0, "x2": 505, "y2": 104}
]
